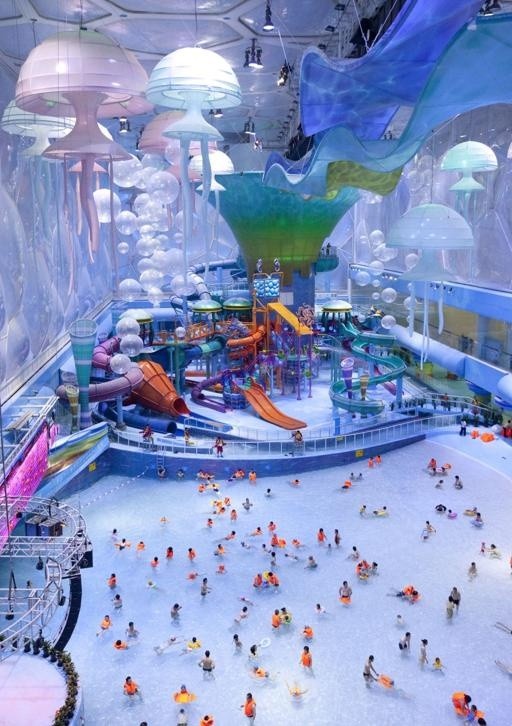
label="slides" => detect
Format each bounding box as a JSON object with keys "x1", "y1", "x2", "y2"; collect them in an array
[
  {"x1": 233, "y1": 377, "x2": 307, "y2": 430},
  {"x1": 329, "y1": 322, "x2": 406, "y2": 416}
]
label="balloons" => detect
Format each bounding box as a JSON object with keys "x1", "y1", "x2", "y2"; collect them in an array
[
  {"x1": 354, "y1": 155, "x2": 435, "y2": 330},
  {"x1": 92, "y1": 139, "x2": 197, "y2": 375}
]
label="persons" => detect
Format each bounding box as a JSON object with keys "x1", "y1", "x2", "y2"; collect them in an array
[
  {"x1": 380, "y1": 345, "x2": 383, "y2": 356},
  {"x1": 326, "y1": 243, "x2": 331, "y2": 256},
  {"x1": 185, "y1": 428, "x2": 194, "y2": 445},
  {"x1": 215, "y1": 380, "x2": 221, "y2": 393},
  {"x1": 386, "y1": 348, "x2": 390, "y2": 356},
  {"x1": 290, "y1": 430, "x2": 303, "y2": 454},
  {"x1": 139, "y1": 424, "x2": 152, "y2": 442},
  {"x1": 458, "y1": 333, "x2": 473, "y2": 354},
  {"x1": 212, "y1": 437, "x2": 225, "y2": 458},
  {"x1": 388, "y1": 131, "x2": 393, "y2": 140},
  {"x1": 28, "y1": 455, "x2": 507, "y2": 726},
  {"x1": 390, "y1": 391, "x2": 512, "y2": 437}
]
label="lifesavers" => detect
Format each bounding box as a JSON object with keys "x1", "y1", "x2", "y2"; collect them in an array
[{"x1": 260, "y1": 637, "x2": 270, "y2": 648}]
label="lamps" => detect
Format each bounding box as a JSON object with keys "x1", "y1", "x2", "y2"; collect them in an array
[
  {"x1": 262, "y1": 0, "x2": 274, "y2": 32},
  {"x1": 277, "y1": 0, "x2": 349, "y2": 142},
  {"x1": 242, "y1": 37, "x2": 264, "y2": 70},
  {"x1": 243, "y1": 116, "x2": 255, "y2": 135},
  {"x1": 209, "y1": 109, "x2": 223, "y2": 117},
  {"x1": 477, "y1": 0, "x2": 502, "y2": 18},
  {"x1": 118, "y1": 116, "x2": 131, "y2": 135}
]
